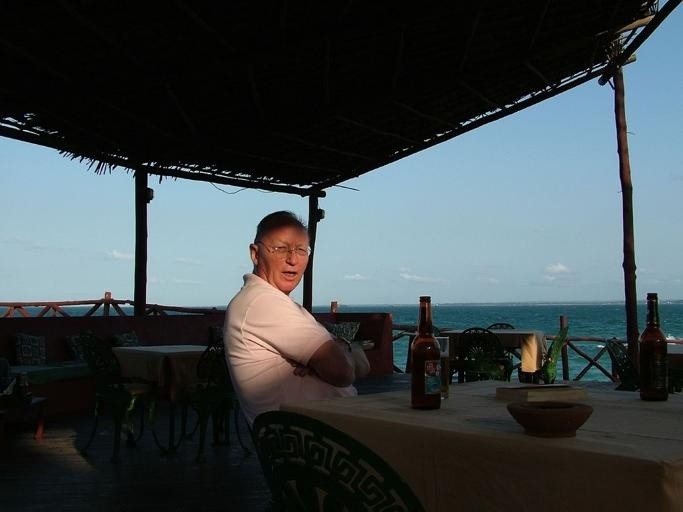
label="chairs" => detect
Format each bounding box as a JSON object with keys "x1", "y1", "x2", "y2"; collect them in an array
[
  {"x1": 457, "y1": 327, "x2": 513, "y2": 384},
  {"x1": 225, "y1": 405, "x2": 426, "y2": 512},
  {"x1": 170, "y1": 334, "x2": 234, "y2": 464},
  {"x1": 92, "y1": 330, "x2": 154, "y2": 448},
  {"x1": 608, "y1": 341, "x2": 631, "y2": 378},
  {"x1": 487, "y1": 323, "x2": 515, "y2": 328}
]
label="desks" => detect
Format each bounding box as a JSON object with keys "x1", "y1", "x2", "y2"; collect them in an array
[
  {"x1": 667, "y1": 341, "x2": 683, "y2": 392},
  {"x1": 111, "y1": 342, "x2": 225, "y2": 452},
  {"x1": 280, "y1": 380, "x2": 683, "y2": 512},
  {"x1": 433, "y1": 330, "x2": 545, "y2": 381}
]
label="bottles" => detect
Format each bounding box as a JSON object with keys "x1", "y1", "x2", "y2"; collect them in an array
[
  {"x1": 410, "y1": 296, "x2": 441, "y2": 410},
  {"x1": 639, "y1": 293, "x2": 669, "y2": 401}
]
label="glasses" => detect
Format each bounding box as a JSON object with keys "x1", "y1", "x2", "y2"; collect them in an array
[{"x1": 256, "y1": 239, "x2": 312, "y2": 257}]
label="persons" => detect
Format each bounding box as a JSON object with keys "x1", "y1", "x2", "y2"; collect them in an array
[{"x1": 222, "y1": 210, "x2": 371, "y2": 439}]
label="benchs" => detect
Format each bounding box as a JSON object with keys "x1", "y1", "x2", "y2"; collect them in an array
[{"x1": 0, "y1": 313, "x2": 393, "y2": 439}]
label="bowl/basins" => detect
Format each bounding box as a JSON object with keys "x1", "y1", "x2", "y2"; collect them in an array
[{"x1": 507, "y1": 401, "x2": 594, "y2": 436}]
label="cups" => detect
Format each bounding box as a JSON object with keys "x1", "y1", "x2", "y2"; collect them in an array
[{"x1": 436, "y1": 336, "x2": 450, "y2": 399}]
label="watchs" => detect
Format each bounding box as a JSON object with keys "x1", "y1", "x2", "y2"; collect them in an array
[{"x1": 338, "y1": 335, "x2": 351, "y2": 353}]
